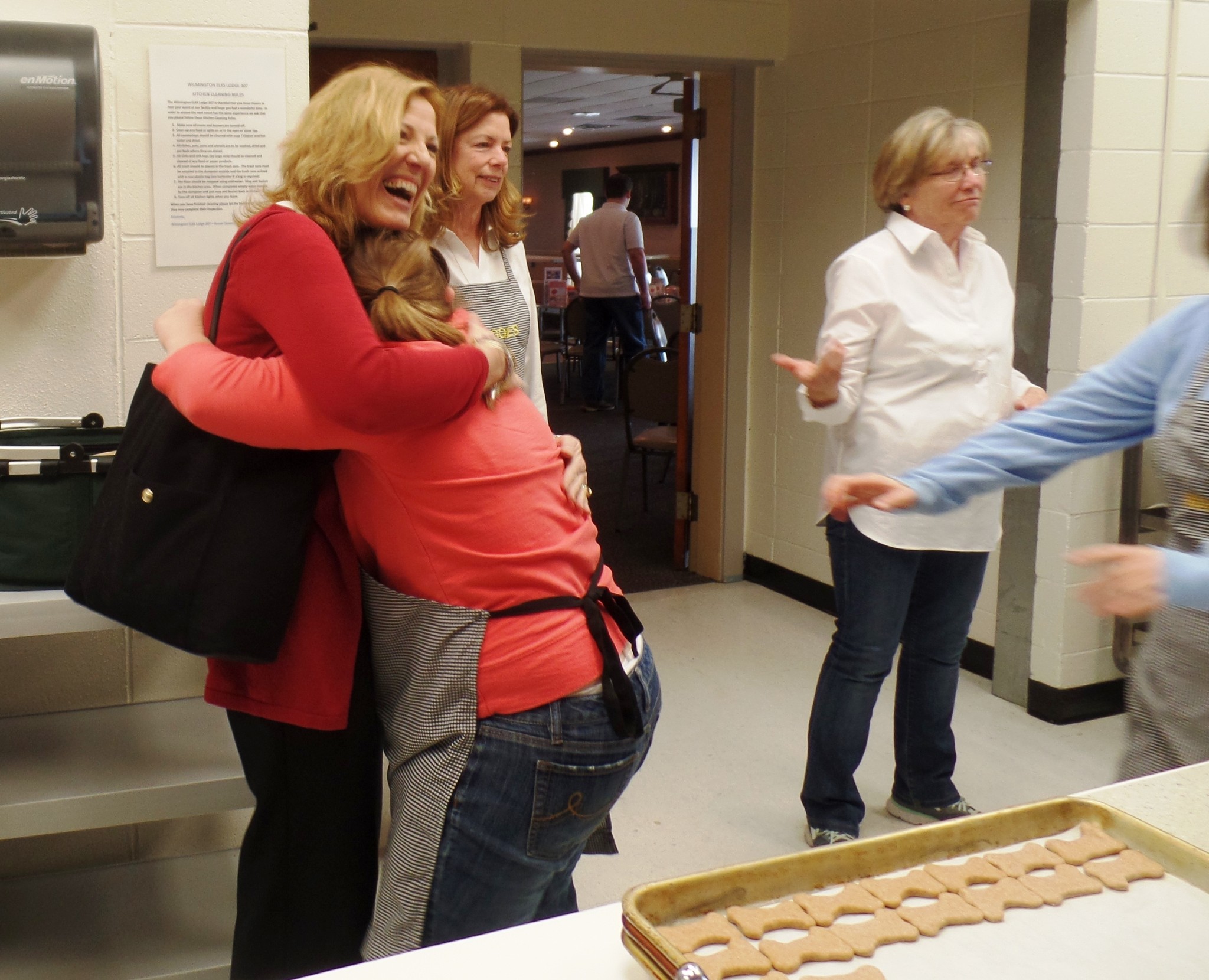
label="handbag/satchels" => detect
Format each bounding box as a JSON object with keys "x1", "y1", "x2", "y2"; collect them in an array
[{"x1": 62, "y1": 214, "x2": 340, "y2": 662}]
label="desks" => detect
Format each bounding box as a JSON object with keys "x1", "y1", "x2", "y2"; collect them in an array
[
  {"x1": 299, "y1": 758, "x2": 1209, "y2": 980},
  {"x1": 536, "y1": 303, "x2": 564, "y2": 340}
]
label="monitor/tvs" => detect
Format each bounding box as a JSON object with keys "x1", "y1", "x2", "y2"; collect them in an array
[{"x1": 0, "y1": 20, "x2": 107, "y2": 254}]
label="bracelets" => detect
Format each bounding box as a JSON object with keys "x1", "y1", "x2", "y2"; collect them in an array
[{"x1": 471, "y1": 335, "x2": 516, "y2": 409}]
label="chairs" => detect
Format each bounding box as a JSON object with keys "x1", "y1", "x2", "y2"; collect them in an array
[
  {"x1": 654, "y1": 265, "x2": 670, "y2": 287},
  {"x1": 621, "y1": 346, "x2": 678, "y2": 512},
  {"x1": 540, "y1": 340, "x2": 563, "y2": 391},
  {"x1": 644, "y1": 295, "x2": 680, "y2": 340},
  {"x1": 644, "y1": 309, "x2": 678, "y2": 426},
  {"x1": 563, "y1": 297, "x2": 624, "y2": 408}
]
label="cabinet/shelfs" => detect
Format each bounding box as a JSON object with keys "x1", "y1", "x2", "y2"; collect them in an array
[{"x1": 0, "y1": 590, "x2": 389, "y2": 980}]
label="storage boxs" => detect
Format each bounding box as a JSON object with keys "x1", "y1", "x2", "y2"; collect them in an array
[{"x1": 0, "y1": 413, "x2": 126, "y2": 593}]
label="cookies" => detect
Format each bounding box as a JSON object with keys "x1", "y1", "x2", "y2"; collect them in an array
[{"x1": 656, "y1": 821, "x2": 1163, "y2": 980}]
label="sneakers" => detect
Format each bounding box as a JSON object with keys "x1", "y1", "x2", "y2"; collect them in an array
[
  {"x1": 889, "y1": 793, "x2": 982, "y2": 826},
  {"x1": 804, "y1": 824, "x2": 856, "y2": 846}
]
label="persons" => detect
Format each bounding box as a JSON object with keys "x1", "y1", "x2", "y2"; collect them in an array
[
  {"x1": 153, "y1": 232, "x2": 660, "y2": 947},
  {"x1": 770, "y1": 108, "x2": 1049, "y2": 848},
  {"x1": 201, "y1": 64, "x2": 514, "y2": 980},
  {"x1": 436, "y1": 86, "x2": 547, "y2": 422},
  {"x1": 561, "y1": 172, "x2": 655, "y2": 422},
  {"x1": 822, "y1": 155, "x2": 1209, "y2": 783}
]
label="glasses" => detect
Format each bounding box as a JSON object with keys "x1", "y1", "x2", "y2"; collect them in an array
[{"x1": 925, "y1": 159, "x2": 994, "y2": 183}]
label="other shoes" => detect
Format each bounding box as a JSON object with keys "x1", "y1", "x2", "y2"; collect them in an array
[{"x1": 582, "y1": 401, "x2": 613, "y2": 412}]
